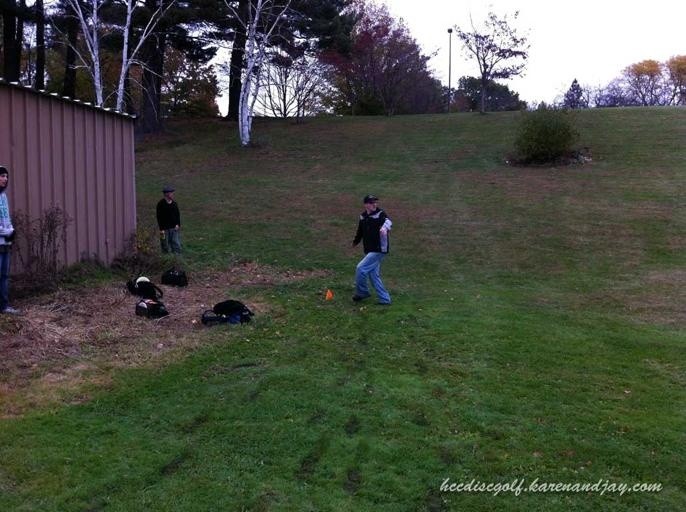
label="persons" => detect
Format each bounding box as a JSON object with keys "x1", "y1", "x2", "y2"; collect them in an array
[
  {"x1": 156, "y1": 188, "x2": 182, "y2": 253},
  {"x1": 0, "y1": 165, "x2": 22, "y2": 314},
  {"x1": 352, "y1": 195, "x2": 393, "y2": 306}
]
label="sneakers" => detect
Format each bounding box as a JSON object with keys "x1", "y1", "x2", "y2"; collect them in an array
[
  {"x1": 353, "y1": 294, "x2": 371, "y2": 301},
  {"x1": 2, "y1": 305, "x2": 20, "y2": 313},
  {"x1": 374, "y1": 299, "x2": 392, "y2": 304}
]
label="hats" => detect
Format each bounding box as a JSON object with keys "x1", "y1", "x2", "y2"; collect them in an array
[
  {"x1": 364, "y1": 194, "x2": 378, "y2": 203},
  {"x1": 162, "y1": 185, "x2": 175, "y2": 192}
]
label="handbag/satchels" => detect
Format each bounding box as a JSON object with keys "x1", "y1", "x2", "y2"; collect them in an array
[
  {"x1": 162, "y1": 267, "x2": 187, "y2": 286},
  {"x1": 212, "y1": 300, "x2": 254, "y2": 321},
  {"x1": 127, "y1": 278, "x2": 156, "y2": 298},
  {"x1": 136, "y1": 300, "x2": 168, "y2": 318}
]
